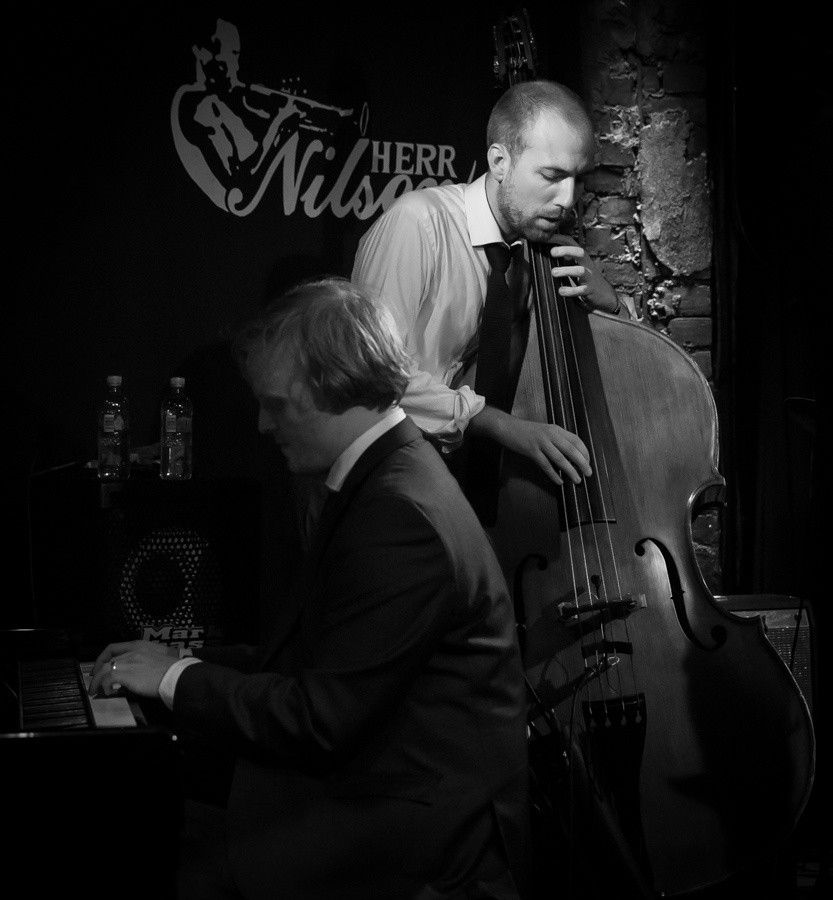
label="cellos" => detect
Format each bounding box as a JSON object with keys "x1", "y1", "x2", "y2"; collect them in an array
[{"x1": 432, "y1": 6, "x2": 818, "y2": 900}]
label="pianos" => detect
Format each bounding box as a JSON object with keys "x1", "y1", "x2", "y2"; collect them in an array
[{"x1": 0, "y1": 620, "x2": 189, "y2": 900}]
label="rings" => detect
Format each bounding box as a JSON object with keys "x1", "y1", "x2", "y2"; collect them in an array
[{"x1": 111, "y1": 661, "x2": 117, "y2": 671}]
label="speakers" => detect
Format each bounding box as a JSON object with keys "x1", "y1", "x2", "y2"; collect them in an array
[{"x1": 711, "y1": 593, "x2": 817, "y2": 723}]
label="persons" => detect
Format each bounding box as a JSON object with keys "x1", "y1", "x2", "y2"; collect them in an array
[
  {"x1": 352, "y1": 81, "x2": 633, "y2": 518},
  {"x1": 86, "y1": 280, "x2": 528, "y2": 900}
]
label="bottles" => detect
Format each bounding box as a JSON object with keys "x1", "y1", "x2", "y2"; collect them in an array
[
  {"x1": 95, "y1": 377, "x2": 129, "y2": 482},
  {"x1": 159, "y1": 378, "x2": 194, "y2": 479}
]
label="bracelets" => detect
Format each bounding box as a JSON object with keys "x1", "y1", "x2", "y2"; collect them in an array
[{"x1": 611, "y1": 294, "x2": 621, "y2": 314}]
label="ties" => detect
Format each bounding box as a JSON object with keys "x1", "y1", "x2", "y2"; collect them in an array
[{"x1": 465, "y1": 242, "x2": 513, "y2": 531}]
